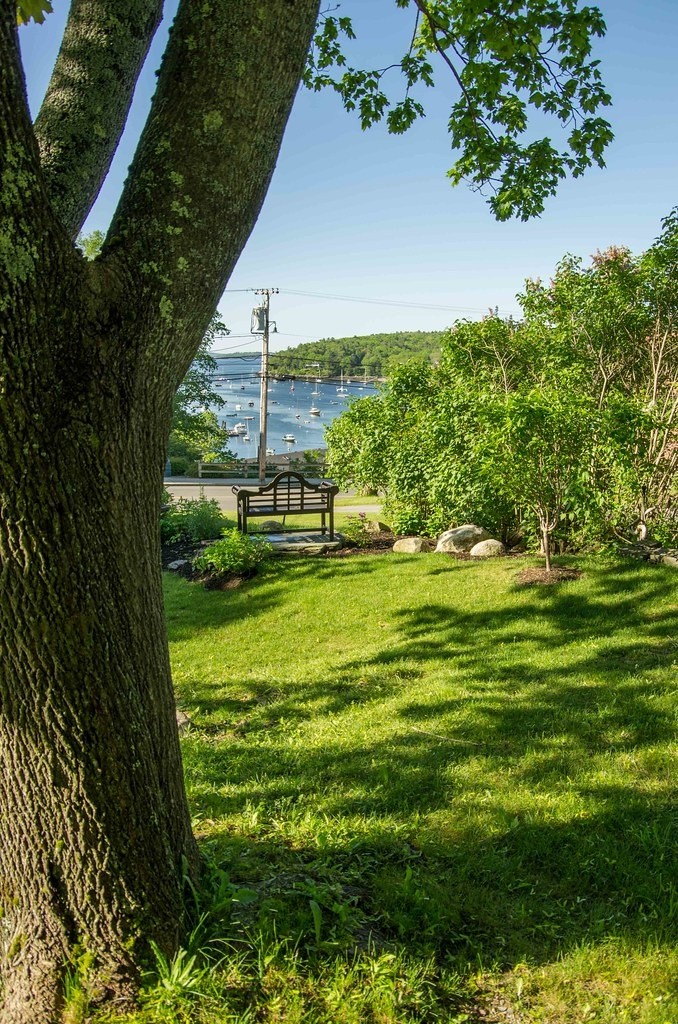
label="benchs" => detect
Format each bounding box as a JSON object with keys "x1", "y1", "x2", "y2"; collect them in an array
[{"x1": 232, "y1": 470, "x2": 339, "y2": 541}]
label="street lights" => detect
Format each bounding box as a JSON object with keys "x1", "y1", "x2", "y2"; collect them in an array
[{"x1": 259, "y1": 320, "x2": 279, "y2": 483}]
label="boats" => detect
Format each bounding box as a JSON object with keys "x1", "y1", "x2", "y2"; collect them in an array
[
  {"x1": 248, "y1": 399, "x2": 254, "y2": 407},
  {"x1": 290, "y1": 380, "x2": 295, "y2": 392},
  {"x1": 215, "y1": 384, "x2": 222, "y2": 387},
  {"x1": 308, "y1": 407, "x2": 320, "y2": 417},
  {"x1": 241, "y1": 385, "x2": 245, "y2": 389},
  {"x1": 281, "y1": 434, "x2": 295, "y2": 442},
  {"x1": 267, "y1": 448, "x2": 276, "y2": 454},
  {"x1": 220, "y1": 421, "x2": 255, "y2": 442},
  {"x1": 310, "y1": 383, "x2": 319, "y2": 396},
  {"x1": 226, "y1": 414, "x2": 238, "y2": 417},
  {"x1": 250, "y1": 380, "x2": 258, "y2": 384}
]
